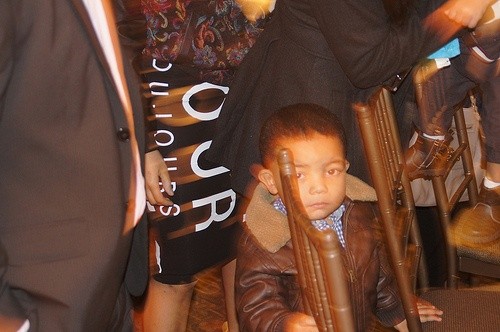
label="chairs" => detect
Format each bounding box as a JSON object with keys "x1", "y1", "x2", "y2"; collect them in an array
[
  {"x1": 351, "y1": 82, "x2": 500, "y2": 331},
  {"x1": 276, "y1": 145, "x2": 358, "y2": 331},
  {"x1": 410, "y1": 49, "x2": 499, "y2": 288}
]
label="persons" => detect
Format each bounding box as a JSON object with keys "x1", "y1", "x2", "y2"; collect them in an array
[
  {"x1": 444, "y1": 0, "x2": 500, "y2": 185},
  {"x1": 225, "y1": 1, "x2": 499, "y2": 232},
  {"x1": 111, "y1": 0, "x2": 275, "y2": 332},
  {"x1": 234, "y1": 104, "x2": 445, "y2": 332},
  {"x1": 0, "y1": 0, "x2": 150, "y2": 332}
]
